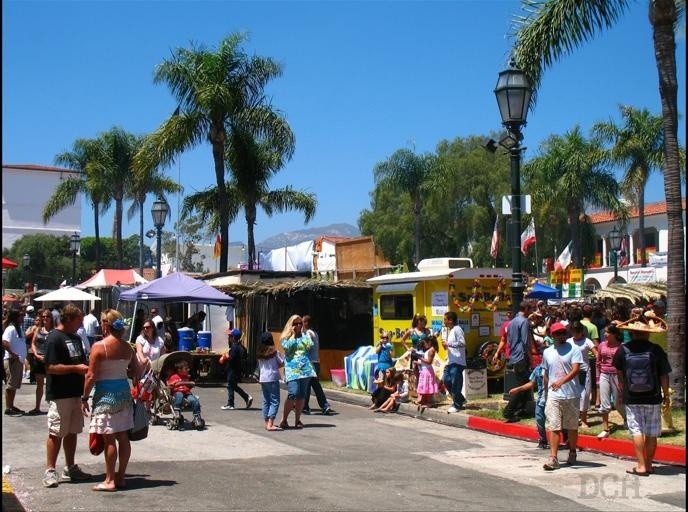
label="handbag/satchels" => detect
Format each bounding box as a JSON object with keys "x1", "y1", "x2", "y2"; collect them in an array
[
  {"x1": 579, "y1": 371, "x2": 586, "y2": 384},
  {"x1": 90, "y1": 433, "x2": 104, "y2": 456},
  {"x1": 128, "y1": 352, "x2": 148, "y2": 378},
  {"x1": 132, "y1": 374, "x2": 156, "y2": 400},
  {"x1": 513, "y1": 359, "x2": 530, "y2": 374},
  {"x1": 129, "y1": 398, "x2": 148, "y2": 441}
]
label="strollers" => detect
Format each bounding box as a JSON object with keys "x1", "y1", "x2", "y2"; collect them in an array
[{"x1": 143, "y1": 350, "x2": 205, "y2": 431}]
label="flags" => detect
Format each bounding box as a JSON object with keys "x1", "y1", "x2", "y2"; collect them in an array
[
  {"x1": 489, "y1": 219, "x2": 499, "y2": 258},
  {"x1": 212, "y1": 235, "x2": 221, "y2": 260},
  {"x1": 554, "y1": 239, "x2": 573, "y2": 273},
  {"x1": 617, "y1": 233, "x2": 630, "y2": 268},
  {"x1": 520, "y1": 219, "x2": 536, "y2": 257}
]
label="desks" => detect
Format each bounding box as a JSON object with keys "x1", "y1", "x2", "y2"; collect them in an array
[{"x1": 189, "y1": 349, "x2": 223, "y2": 382}]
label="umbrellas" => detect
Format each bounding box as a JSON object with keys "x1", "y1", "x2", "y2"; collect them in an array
[{"x1": 32, "y1": 285, "x2": 102, "y2": 302}]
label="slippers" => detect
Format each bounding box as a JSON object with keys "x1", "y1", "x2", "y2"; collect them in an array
[
  {"x1": 93, "y1": 482, "x2": 128, "y2": 491},
  {"x1": 626, "y1": 466, "x2": 654, "y2": 476}
]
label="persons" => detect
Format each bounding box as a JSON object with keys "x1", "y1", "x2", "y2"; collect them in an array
[
  {"x1": 441, "y1": 311, "x2": 467, "y2": 414},
  {"x1": 150, "y1": 308, "x2": 164, "y2": 340},
  {"x1": 256, "y1": 332, "x2": 284, "y2": 430},
  {"x1": 82, "y1": 309, "x2": 99, "y2": 347},
  {"x1": 42, "y1": 303, "x2": 91, "y2": 488},
  {"x1": 81, "y1": 308, "x2": 141, "y2": 492},
  {"x1": 75, "y1": 328, "x2": 90, "y2": 355},
  {"x1": 280, "y1": 314, "x2": 318, "y2": 429},
  {"x1": 220, "y1": 328, "x2": 253, "y2": 411},
  {"x1": 493, "y1": 300, "x2": 669, "y2": 476},
  {"x1": 369, "y1": 312, "x2": 441, "y2": 414},
  {"x1": 302, "y1": 315, "x2": 336, "y2": 416},
  {"x1": 132, "y1": 318, "x2": 166, "y2": 414},
  {"x1": 165, "y1": 359, "x2": 202, "y2": 427},
  {"x1": 1, "y1": 302, "x2": 65, "y2": 417}
]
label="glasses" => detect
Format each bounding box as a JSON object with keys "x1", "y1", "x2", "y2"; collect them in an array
[{"x1": 141, "y1": 326, "x2": 152, "y2": 329}]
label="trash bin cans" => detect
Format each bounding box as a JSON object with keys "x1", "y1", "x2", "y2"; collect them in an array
[{"x1": 461, "y1": 356, "x2": 488, "y2": 401}]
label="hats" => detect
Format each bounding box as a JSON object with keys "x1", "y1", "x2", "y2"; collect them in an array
[
  {"x1": 550, "y1": 322, "x2": 567, "y2": 334},
  {"x1": 26, "y1": 306, "x2": 34, "y2": 312},
  {"x1": 225, "y1": 329, "x2": 241, "y2": 338}
]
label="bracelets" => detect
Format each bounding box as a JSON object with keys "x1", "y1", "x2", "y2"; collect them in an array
[{"x1": 80, "y1": 395, "x2": 89, "y2": 401}]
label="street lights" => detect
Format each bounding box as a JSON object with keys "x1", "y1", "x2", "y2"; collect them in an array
[
  {"x1": 606, "y1": 225, "x2": 625, "y2": 284},
  {"x1": 151, "y1": 194, "x2": 169, "y2": 281},
  {"x1": 494, "y1": 57, "x2": 536, "y2": 401},
  {"x1": 2, "y1": 268, "x2": 7, "y2": 295},
  {"x1": 67, "y1": 231, "x2": 81, "y2": 286},
  {"x1": 21, "y1": 253, "x2": 32, "y2": 293},
  {"x1": 257, "y1": 250, "x2": 263, "y2": 270}
]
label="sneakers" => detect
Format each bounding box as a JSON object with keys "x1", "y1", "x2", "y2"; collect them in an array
[
  {"x1": 265, "y1": 420, "x2": 302, "y2": 431},
  {"x1": 503, "y1": 412, "x2": 520, "y2": 421},
  {"x1": 63, "y1": 464, "x2": 92, "y2": 480},
  {"x1": 246, "y1": 395, "x2": 253, "y2": 408},
  {"x1": 447, "y1": 399, "x2": 467, "y2": 413},
  {"x1": 597, "y1": 430, "x2": 609, "y2": 438},
  {"x1": 538, "y1": 436, "x2": 577, "y2": 470},
  {"x1": 294, "y1": 406, "x2": 330, "y2": 415},
  {"x1": 43, "y1": 468, "x2": 59, "y2": 487},
  {"x1": 5, "y1": 407, "x2": 40, "y2": 417},
  {"x1": 221, "y1": 405, "x2": 235, "y2": 409}
]
